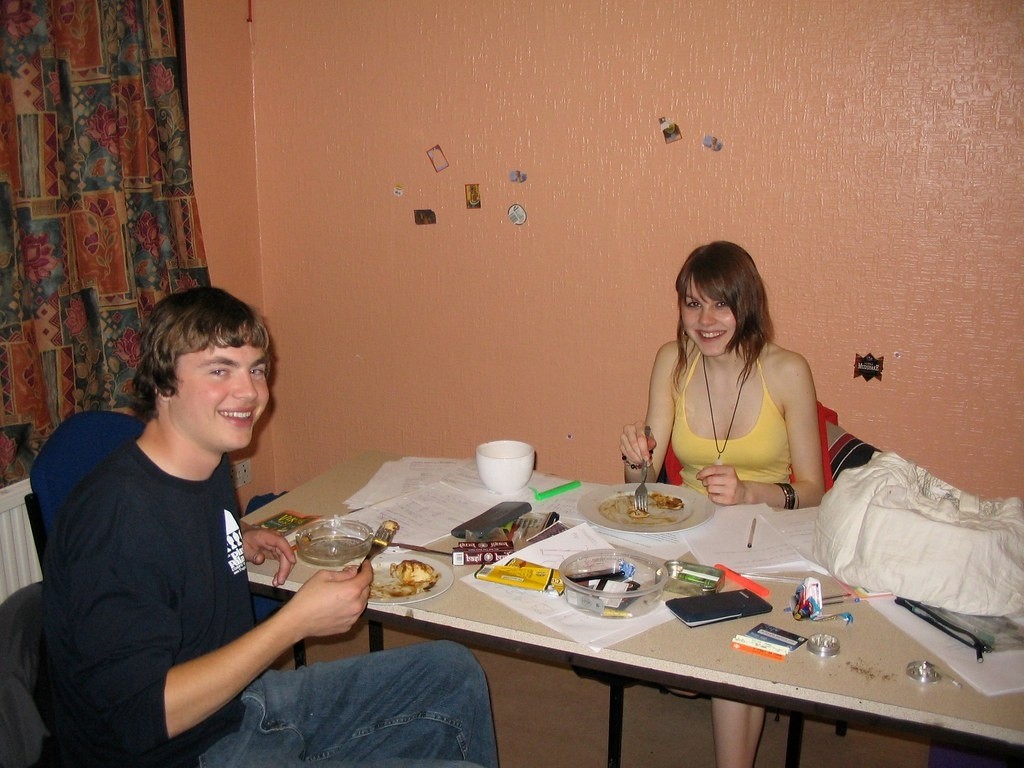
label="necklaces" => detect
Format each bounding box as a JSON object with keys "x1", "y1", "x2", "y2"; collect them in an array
[{"x1": 703, "y1": 356, "x2": 747, "y2": 464}]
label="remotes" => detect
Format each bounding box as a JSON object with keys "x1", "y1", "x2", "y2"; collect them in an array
[{"x1": 451, "y1": 502, "x2": 531, "y2": 539}]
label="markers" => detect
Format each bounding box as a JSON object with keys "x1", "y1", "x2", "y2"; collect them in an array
[
  {"x1": 535, "y1": 481, "x2": 581, "y2": 500},
  {"x1": 678, "y1": 573, "x2": 716, "y2": 586}
]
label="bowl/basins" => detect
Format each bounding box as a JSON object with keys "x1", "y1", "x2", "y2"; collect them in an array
[
  {"x1": 476, "y1": 440, "x2": 534, "y2": 494},
  {"x1": 559, "y1": 548, "x2": 668, "y2": 618}
]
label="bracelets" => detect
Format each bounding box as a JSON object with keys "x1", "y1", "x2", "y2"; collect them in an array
[
  {"x1": 775, "y1": 483, "x2": 800, "y2": 510},
  {"x1": 621, "y1": 450, "x2": 654, "y2": 470}
]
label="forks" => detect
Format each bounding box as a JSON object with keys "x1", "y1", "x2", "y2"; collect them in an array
[
  {"x1": 357, "y1": 521, "x2": 398, "y2": 575},
  {"x1": 634, "y1": 426, "x2": 651, "y2": 513}
]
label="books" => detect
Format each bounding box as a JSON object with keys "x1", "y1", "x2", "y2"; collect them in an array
[{"x1": 665, "y1": 589, "x2": 773, "y2": 628}]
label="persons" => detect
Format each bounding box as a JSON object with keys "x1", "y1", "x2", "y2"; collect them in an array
[
  {"x1": 619, "y1": 241, "x2": 826, "y2": 768},
  {"x1": 36, "y1": 286, "x2": 497, "y2": 768}
]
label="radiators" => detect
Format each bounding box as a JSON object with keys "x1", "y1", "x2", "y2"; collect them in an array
[{"x1": 0, "y1": 478, "x2": 42, "y2": 608}]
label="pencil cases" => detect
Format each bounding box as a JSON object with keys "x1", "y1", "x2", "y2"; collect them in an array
[{"x1": 894, "y1": 597, "x2": 1024, "y2": 663}]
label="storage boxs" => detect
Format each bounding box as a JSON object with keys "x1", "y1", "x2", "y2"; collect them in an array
[
  {"x1": 655, "y1": 560, "x2": 726, "y2": 595},
  {"x1": 558, "y1": 548, "x2": 668, "y2": 621}
]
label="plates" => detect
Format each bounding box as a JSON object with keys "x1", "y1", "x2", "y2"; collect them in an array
[
  {"x1": 578, "y1": 483, "x2": 715, "y2": 533},
  {"x1": 343, "y1": 554, "x2": 454, "y2": 605}
]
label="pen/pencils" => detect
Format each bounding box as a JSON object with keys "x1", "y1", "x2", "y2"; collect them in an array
[
  {"x1": 714, "y1": 564, "x2": 769, "y2": 597},
  {"x1": 747, "y1": 518, "x2": 756, "y2": 548}
]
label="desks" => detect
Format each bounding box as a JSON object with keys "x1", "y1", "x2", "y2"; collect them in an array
[{"x1": 240, "y1": 452, "x2": 1024, "y2": 768}]
